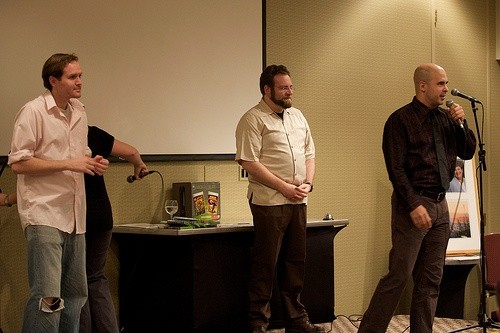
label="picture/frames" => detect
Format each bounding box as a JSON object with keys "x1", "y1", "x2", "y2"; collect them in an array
[{"x1": 443, "y1": 155, "x2": 481, "y2": 256}]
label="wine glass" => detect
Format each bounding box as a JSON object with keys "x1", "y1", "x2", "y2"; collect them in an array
[{"x1": 165, "y1": 200, "x2": 178, "y2": 220}]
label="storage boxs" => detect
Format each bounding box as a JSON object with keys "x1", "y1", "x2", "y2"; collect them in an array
[{"x1": 172, "y1": 181, "x2": 222, "y2": 222}]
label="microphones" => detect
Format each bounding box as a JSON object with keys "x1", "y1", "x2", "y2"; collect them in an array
[
  {"x1": 127, "y1": 170, "x2": 155, "y2": 183},
  {"x1": 451, "y1": 88, "x2": 482, "y2": 104},
  {"x1": 446, "y1": 99, "x2": 466, "y2": 135}
]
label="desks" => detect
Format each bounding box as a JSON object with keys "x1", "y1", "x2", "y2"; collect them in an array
[
  {"x1": 112, "y1": 219, "x2": 349, "y2": 333},
  {"x1": 435, "y1": 255, "x2": 480, "y2": 319}
]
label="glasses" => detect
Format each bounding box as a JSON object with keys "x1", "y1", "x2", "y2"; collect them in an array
[{"x1": 269, "y1": 85, "x2": 296, "y2": 92}]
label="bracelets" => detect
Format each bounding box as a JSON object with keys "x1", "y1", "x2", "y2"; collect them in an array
[
  {"x1": 305, "y1": 182, "x2": 314, "y2": 192},
  {"x1": 4, "y1": 194, "x2": 12, "y2": 208}
]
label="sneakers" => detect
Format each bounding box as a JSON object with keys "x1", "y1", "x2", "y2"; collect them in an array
[
  {"x1": 284, "y1": 317, "x2": 325, "y2": 333},
  {"x1": 251, "y1": 327, "x2": 266, "y2": 333}
]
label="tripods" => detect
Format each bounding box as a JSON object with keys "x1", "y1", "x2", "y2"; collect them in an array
[{"x1": 449, "y1": 101, "x2": 500, "y2": 333}]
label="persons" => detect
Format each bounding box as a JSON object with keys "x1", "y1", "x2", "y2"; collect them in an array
[
  {"x1": 8, "y1": 53, "x2": 109, "y2": 333},
  {"x1": 447, "y1": 161, "x2": 466, "y2": 192},
  {"x1": 0, "y1": 125, "x2": 148, "y2": 333},
  {"x1": 357, "y1": 62, "x2": 477, "y2": 333},
  {"x1": 233, "y1": 65, "x2": 325, "y2": 333}
]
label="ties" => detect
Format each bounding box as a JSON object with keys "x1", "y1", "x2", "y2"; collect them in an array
[{"x1": 429, "y1": 109, "x2": 450, "y2": 191}]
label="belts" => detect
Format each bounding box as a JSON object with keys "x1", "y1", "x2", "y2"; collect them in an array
[{"x1": 414, "y1": 186, "x2": 445, "y2": 202}]
